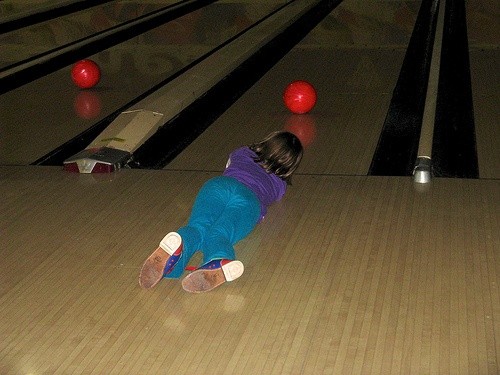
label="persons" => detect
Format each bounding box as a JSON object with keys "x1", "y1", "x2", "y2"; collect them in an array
[{"x1": 137, "y1": 130, "x2": 304, "y2": 294}]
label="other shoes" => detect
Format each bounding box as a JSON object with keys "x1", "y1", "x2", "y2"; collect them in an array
[
  {"x1": 182, "y1": 259, "x2": 245, "y2": 293},
  {"x1": 139, "y1": 231, "x2": 183, "y2": 289}
]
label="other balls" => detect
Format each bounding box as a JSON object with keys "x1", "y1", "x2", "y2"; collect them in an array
[
  {"x1": 72, "y1": 60, "x2": 99, "y2": 89},
  {"x1": 282, "y1": 80, "x2": 315, "y2": 114}
]
